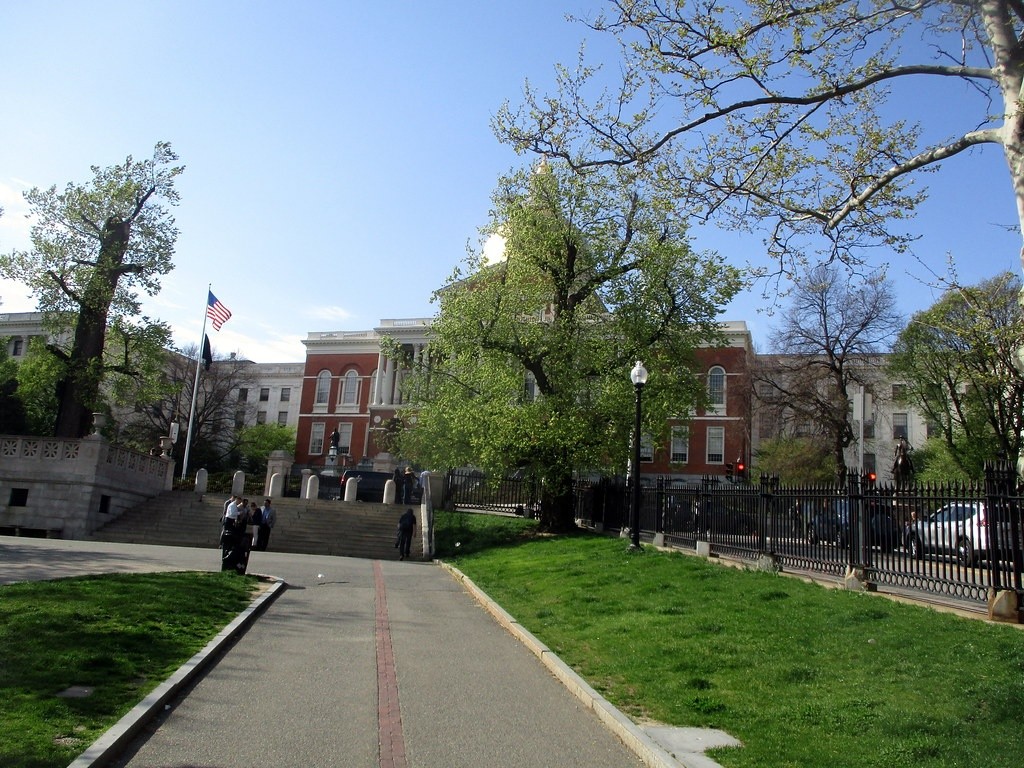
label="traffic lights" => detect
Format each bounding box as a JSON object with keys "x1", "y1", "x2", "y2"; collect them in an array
[
  {"x1": 737, "y1": 462, "x2": 744, "y2": 477},
  {"x1": 725, "y1": 463, "x2": 733, "y2": 482}
]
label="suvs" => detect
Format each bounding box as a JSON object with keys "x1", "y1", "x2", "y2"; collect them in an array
[{"x1": 901, "y1": 500, "x2": 1024, "y2": 569}]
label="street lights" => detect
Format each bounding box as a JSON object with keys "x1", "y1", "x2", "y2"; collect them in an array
[{"x1": 626, "y1": 359, "x2": 648, "y2": 550}]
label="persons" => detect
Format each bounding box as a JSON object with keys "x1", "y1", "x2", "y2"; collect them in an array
[
  {"x1": 392, "y1": 469, "x2": 404, "y2": 504},
  {"x1": 218, "y1": 496, "x2": 275, "y2": 550},
  {"x1": 395, "y1": 508, "x2": 418, "y2": 560},
  {"x1": 402, "y1": 467, "x2": 421, "y2": 505},
  {"x1": 328, "y1": 427, "x2": 341, "y2": 446}
]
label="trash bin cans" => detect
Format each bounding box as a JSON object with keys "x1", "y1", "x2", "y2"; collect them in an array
[{"x1": 221, "y1": 531, "x2": 253, "y2": 574}]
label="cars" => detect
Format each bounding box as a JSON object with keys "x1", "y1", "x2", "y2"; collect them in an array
[
  {"x1": 666, "y1": 501, "x2": 756, "y2": 538},
  {"x1": 806, "y1": 501, "x2": 898, "y2": 556}
]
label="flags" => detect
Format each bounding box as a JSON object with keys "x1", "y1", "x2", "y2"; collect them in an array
[
  {"x1": 201, "y1": 334, "x2": 213, "y2": 371},
  {"x1": 206, "y1": 291, "x2": 232, "y2": 331}
]
